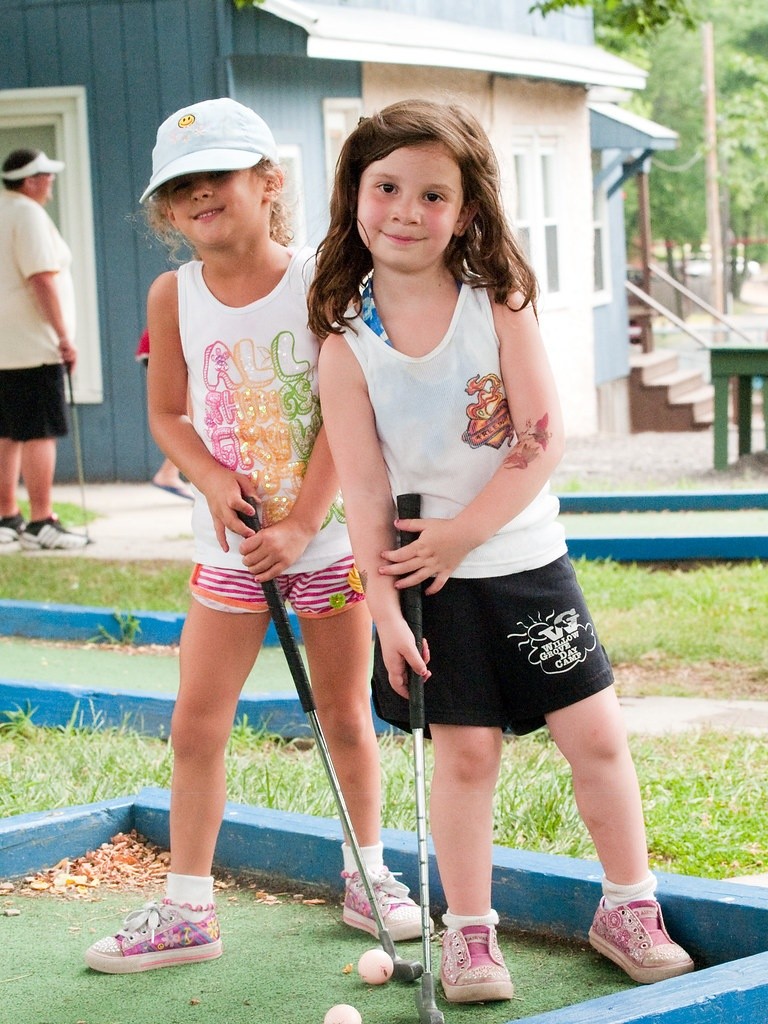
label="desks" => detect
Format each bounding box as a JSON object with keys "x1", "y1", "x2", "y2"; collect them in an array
[{"x1": 704, "y1": 346, "x2": 768, "y2": 472}]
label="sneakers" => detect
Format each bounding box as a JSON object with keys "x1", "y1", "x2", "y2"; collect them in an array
[
  {"x1": 588, "y1": 895, "x2": 692, "y2": 983},
  {"x1": 343, "y1": 866, "x2": 434, "y2": 942},
  {"x1": 0, "y1": 513, "x2": 28, "y2": 555},
  {"x1": 440, "y1": 921, "x2": 516, "y2": 1003},
  {"x1": 23, "y1": 515, "x2": 91, "y2": 550},
  {"x1": 86, "y1": 901, "x2": 221, "y2": 973}
]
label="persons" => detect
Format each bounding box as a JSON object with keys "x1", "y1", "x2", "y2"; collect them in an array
[
  {"x1": 82, "y1": 95, "x2": 437, "y2": 973},
  {"x1": 135, "y1": 329, "x2": 195, "y2": 500},
  {"x1": 299, "y1": 96, "x2": 694, "y2": 1003},
  {"x1": 0, "y1": 147, "x2": 95, "y2": 550}
]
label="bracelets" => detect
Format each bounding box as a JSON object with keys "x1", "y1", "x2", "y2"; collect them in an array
[{"x1": 58, "y1": 334, "x2": 72, "y2": 341}]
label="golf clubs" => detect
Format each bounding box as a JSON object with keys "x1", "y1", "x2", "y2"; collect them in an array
[
  {"x1": 397, "y1": 493, "x2": 445, "y2": 1023},
  {"x1": 241, "y1": 498, "x2": 423, "y2": 986},
  {"x1": 65, "y1": 366, "x2": 94, "y2": 547}
]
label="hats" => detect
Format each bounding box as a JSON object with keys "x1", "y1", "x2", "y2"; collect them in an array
[
  {"x1": 0, "y1": 151, "x2": 65, "y2": 180},
  {"x1": 139, "y1": 97, "x2": 281, "y2": 206}
]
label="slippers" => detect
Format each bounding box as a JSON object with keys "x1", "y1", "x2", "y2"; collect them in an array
[{"x1": 152, "y1": 476, "x2": 194, "y2": 499}]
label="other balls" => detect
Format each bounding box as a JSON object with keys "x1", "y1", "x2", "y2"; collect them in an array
[
  {"x1": 358, "y1": 948, "x2": 393, "y2": 987},
  {"x1": 322, "y1": 1003, "x2": 365, "y2": 1024}
]
